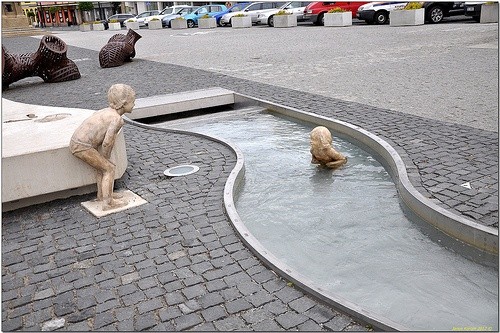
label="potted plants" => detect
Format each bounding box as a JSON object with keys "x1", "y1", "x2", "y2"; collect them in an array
[
  {"x1": 148, "y1": 18, "x2": 163, "y2": 29},
  {"x1": 107, "y1": 19, "x2": 122, "y2": 30},
  {"x1": 324, "y1": 8, "x2": 353, "y2": 26},
  {"x1": 231, "y1": 14, "x2": 253, "y2": 28},
  {"x1": 198, "y1": 14, "x2": 216, "y2": 29},
  {"x1": 480, "y1": 2, "x2": 499, "y2": 24},
  {"x1": 92, "y1": 20, "x2": 104, "y2": 31},
  {"x1": 389, "y1": 1, "x2": 425, "y2": 26},
  {"x1": 171, "y1": 16, "x2": 188, "y2": 28},
  {"x1": 125, "y1": 19, "x2": 140, "y2": 30},
  {"x1": 79, "y1": 21, "x2": 91, "y2": 32},
  {"x1": 273, "y1": 9, "x2": 298, "y2": 28}
]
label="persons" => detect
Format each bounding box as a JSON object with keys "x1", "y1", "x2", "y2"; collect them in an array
[
  {"x1": 226, "y1": 2, "x2": 232, "y2": 9},
  {"x1": 35, "y1": 9, "x2": 40, "y2": 27},
  {"x1": 70, "y1": 83, "x2": 136, "y2": 210},
  {"x1": 40, "y1": 8, "x2": 46, "y2": 28},
  {"x1": 309, "y1": 126, "x2": 347, "y2": 168},
  {"x1": 25, "y1": 8, "x2": 35, "y2": 25},
  {"x1": 105, "y1": 19, "x2": 110, "y2": 30},
  {"x1": 4, "y1": 5, "x2": 8, "y2": 12}
]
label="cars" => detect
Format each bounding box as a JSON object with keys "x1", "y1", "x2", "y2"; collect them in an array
[
  {"x1": 421, "y1": 1, "x2": 465, "y2": 24},
  {"x1": 221, "y1": 2, "x2": 275, "y2": 24},
  {"x1": 101, "y1": 14, "x2": 134, "y2": 30},
  {"x1": 303, "y1": 1, "x2": 371, "y2": 26},
  {"x1": 212, "y1": 3, "x2": 255, "y2": 27},
  {"x1": 256, "y1": 1, "x2": 311, "y2": 27},
  {"x1": 123, "y1": 10, "x2": 160, "y2": 27},
  {"x1": 356, "y1": 1, "x2": 424, "y2": 24},
  {"x1": 144, "y1": 5, "x2": 188, "y2": 24},
  {"x1": 464, "y1": 1, "x2": 500, "y2": 22},
  {"x1": 161, "y1": 6, "x2": 200, "y2": 27},
  {"x1": 185, "y1": 5, "x2": 228, "y2": 28}
]
